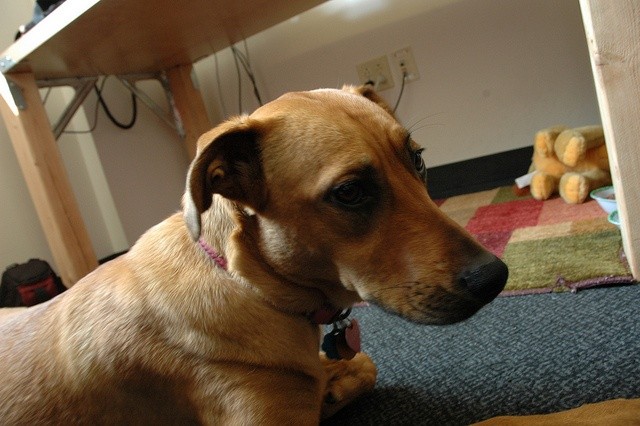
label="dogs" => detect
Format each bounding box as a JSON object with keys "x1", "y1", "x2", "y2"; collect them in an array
[{"x1": 0, "y1": 86, "x2": 509, "y2": 426}]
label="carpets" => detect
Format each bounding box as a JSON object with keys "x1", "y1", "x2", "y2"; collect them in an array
[
  {"x1": 319, "y1": 285, "x2": 640, "y2": 426},
  {"x1": 435, "y1": 187, "x2": 638, "y2": 298}
]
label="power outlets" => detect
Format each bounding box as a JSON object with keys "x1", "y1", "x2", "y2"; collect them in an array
[{"x1": 355, "y1": 56, "x2": 394, "y2": 94}]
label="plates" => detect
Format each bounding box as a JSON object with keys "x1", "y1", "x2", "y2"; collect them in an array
[{"x1": 607, "y1": 209, "x2": 620, "y2": 230}]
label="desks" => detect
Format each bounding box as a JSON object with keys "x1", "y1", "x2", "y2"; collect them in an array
[{"x1": 1, "y1": 2, "x2": 639, "y2": 289}]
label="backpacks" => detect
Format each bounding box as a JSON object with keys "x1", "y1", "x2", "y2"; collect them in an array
[{"x1": 1, "y1": 258, "x2": 65, "y2": 308}]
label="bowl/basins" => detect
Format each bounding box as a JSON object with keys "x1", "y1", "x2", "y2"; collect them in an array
[{"x1": 589, "y1": 185, "x2": 618, "y2": 214}]
